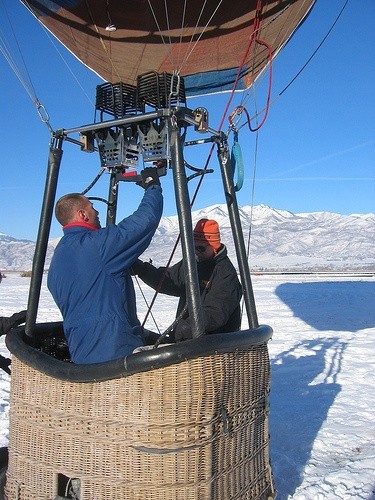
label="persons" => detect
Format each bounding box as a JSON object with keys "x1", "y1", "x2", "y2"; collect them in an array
[
  {"x1": 47, "y1": 167, "x2": 163, "y2": 365},
  {"x1": 127, "y1": 219, "x2": 243, "y2": 343}
]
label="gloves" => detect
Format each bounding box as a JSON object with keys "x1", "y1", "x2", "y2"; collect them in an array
[
  {"x1": 136, "y1": 167, "x2": 160, "y2": 189},
  {"x1": 3, "y1": 309, "x2": 27, "y2": 334},
  {"x1": 170, "y1": 320, "x2": 193, "y2": 342},
  {"x1": 0, "y1": 355, "x2": 11, "y2": 375},
  {"x1": 129, "y1": 259, "x2": 143, "y2": 276}
]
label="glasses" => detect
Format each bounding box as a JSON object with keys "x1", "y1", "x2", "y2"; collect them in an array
[{"x1": 195, "y1": 244, "x2": 211, "y2": 252}]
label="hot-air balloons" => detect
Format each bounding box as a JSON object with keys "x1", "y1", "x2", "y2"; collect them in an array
[{"x1": 0, "y1": 1, "x2": 350, "y2": 500}]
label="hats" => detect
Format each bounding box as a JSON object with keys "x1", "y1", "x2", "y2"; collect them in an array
[{"x1": 193, "y1": 218, "x2": 221, "y2": 249}]
